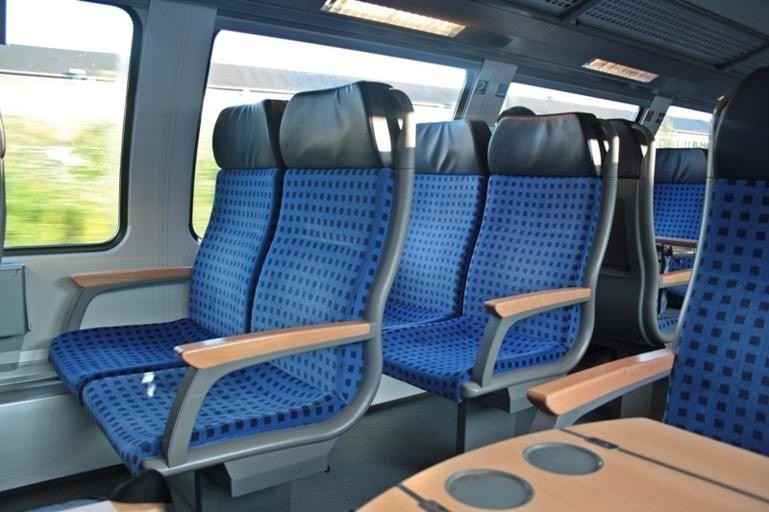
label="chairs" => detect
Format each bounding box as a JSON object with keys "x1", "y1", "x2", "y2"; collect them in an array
[
  {"x1": 356, "y1": 64, "x2": 769, "y2": 512},
  {"x1": 47, "y1": 80, "x2": 417, "y2": 511},
  {"x1": 384, "y1": 112, "x2": 620, "y2": 456},
  {"x1": 596, "y1": 117, "x2": 710, "y2": 420}
]
